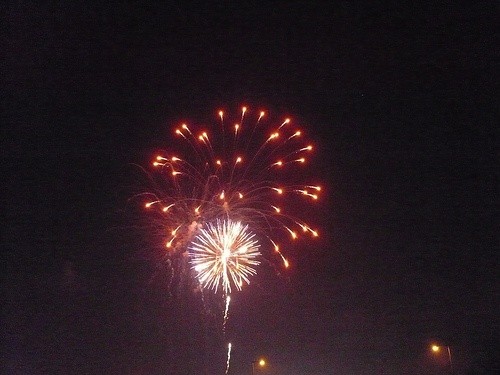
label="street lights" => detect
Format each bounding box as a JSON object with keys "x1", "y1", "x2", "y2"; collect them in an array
[
  {"x1": 432, "y1": 342, "x2": 454, "y2": 375},
  {"x1": 251, "y1": 359, "x2": 265, "y2": 375}
]
